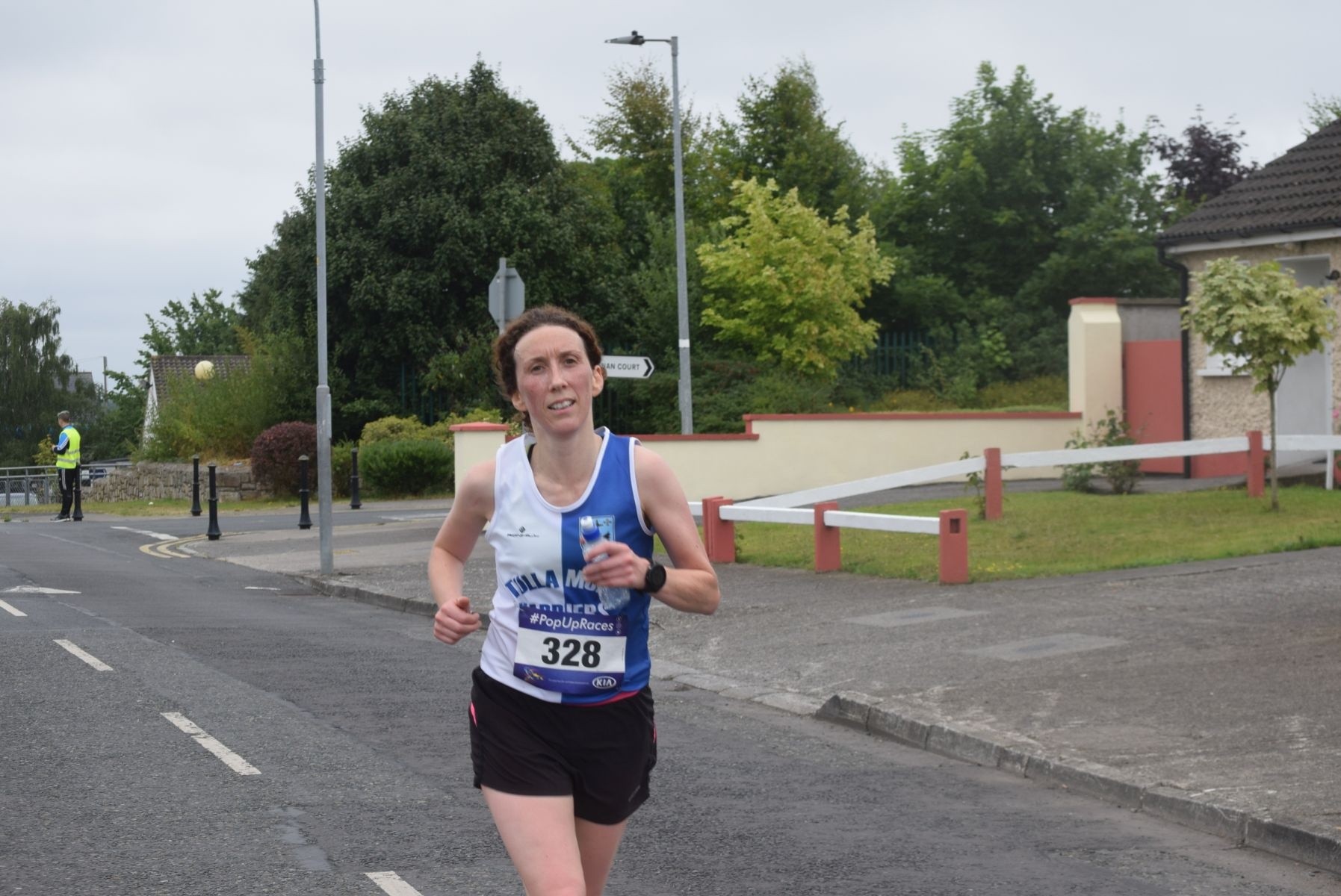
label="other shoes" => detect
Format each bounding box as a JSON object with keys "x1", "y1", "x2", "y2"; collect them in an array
[{"x1": 50, "y1": 514, "x2": 70, "y2": 523}]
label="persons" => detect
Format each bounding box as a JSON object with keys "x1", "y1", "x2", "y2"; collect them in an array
[
  {"x1": 51, "y1": 411, "x2": 81, "y2": 522},
  {"x1": 426, "y1": 305, "x2": 721, "y2": 896}
]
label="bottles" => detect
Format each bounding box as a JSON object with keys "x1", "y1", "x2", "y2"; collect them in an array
[{"x1": 579, "y1": 516, "x2": 630, "y2": 611}]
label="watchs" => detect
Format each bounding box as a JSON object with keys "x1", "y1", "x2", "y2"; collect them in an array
[{"x1": 641, "y1": 558, "x2": 667, "y2": 593}]
